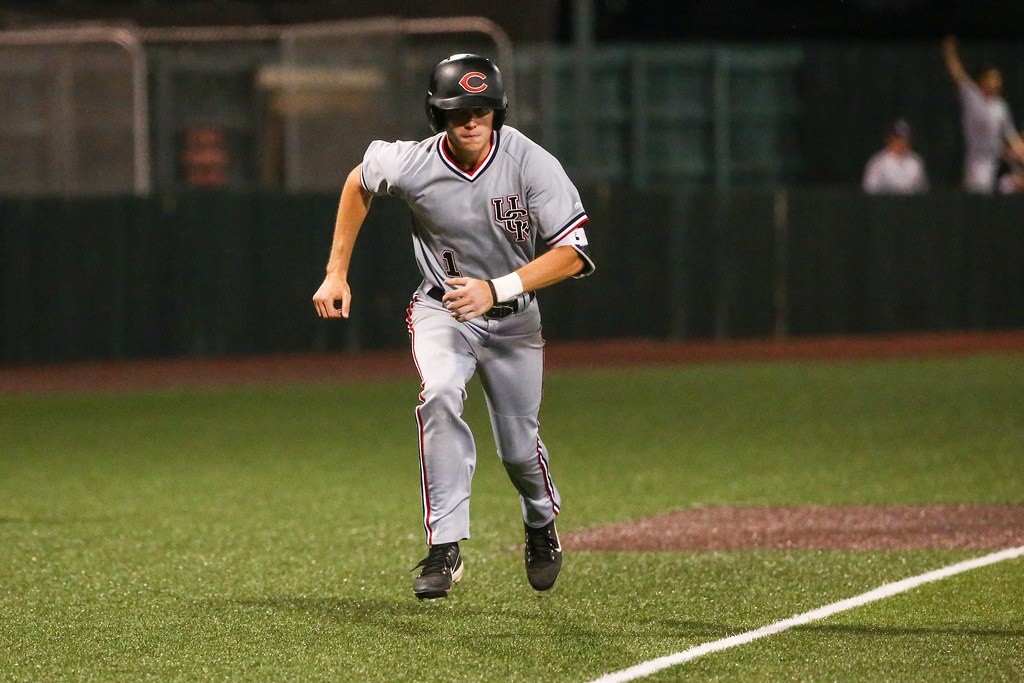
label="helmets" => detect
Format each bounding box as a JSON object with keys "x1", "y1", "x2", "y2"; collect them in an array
[{"x1": 425, "y1": 54, "x2": 508, "y2": 134}]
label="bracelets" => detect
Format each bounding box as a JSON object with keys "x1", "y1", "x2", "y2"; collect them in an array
[{"x1": 485, "y1": 279, "x2": 497, "y2": 305}]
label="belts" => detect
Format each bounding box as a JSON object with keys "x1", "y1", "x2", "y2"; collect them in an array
[{"x1": 427, "y1": 286, "x2": 535, "y2": 320}]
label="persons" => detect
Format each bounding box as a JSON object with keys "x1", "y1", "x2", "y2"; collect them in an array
[
  {"x1": 863, "y1": 32, "x2": 1024, "y2": 196},
  {"x1": 312, "y1": 53, "x2": 596, "y2": 598}
]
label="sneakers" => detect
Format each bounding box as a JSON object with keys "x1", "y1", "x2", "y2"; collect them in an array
[
  {"x1": 411, "y1": 542, "x2": 464, "y2": 600},
  {"x1": 524, "y1": 519, "x2": 562, "y2": 591}
]
